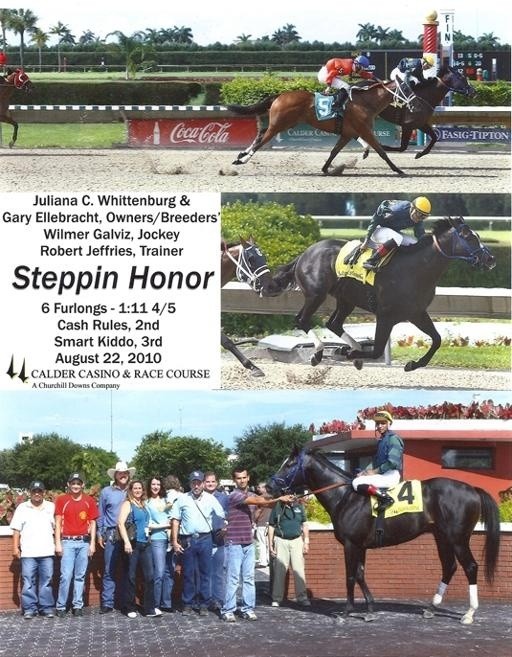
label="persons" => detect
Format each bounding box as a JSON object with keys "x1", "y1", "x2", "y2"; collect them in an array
[
  {"x1": 343, "y1": 194, "x2": 430, "y2": 270},
  {"x1": 8, "y1": 460, "x2": 311, "y2": 623},
  {"x1": 349, "y1": 408, "x2": 404, "y2": 512},
  {"x1": 1, "y1": 47, "x2": 7, "y2": 73},
  {"x1": 318, "y1": 53, "x2": 371, "y2": 101},
  {"x1": 390, "y1": 52, "x2": 435, "y2": 86}
]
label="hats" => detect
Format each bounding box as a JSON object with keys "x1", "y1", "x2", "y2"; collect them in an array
[
  {"x1": 188, "y1": 470, "x2": 205, "y2": 481},
  {"x1": 29, "y1": 462, "x2": 136, "y2": 492}
]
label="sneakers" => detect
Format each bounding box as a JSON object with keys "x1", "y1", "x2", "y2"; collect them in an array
[{"x1": 24, "y1": 600, "x2": 311, "y2": 622}]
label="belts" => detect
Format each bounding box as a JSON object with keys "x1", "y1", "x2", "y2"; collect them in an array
[{"x1": 62, "y1": 536, "x2": 82, "y2": 540}]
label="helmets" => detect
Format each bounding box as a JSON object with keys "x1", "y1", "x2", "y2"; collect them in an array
[
  {"x1": 353, "y1": 55, "x2": 370, "y2": 68},
  {"x1": 412, "y1": 197, "x2": 431, "y2": 216},
  {"x1": 423, "y1": 55, "x2": 434, "y2": 66},
  {"x1": 372, "y1": 410, "x2": 392, "y2": 428}
]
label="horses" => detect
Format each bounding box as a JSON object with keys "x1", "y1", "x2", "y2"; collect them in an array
[
  {"x1": 264, "y1": 442, "x2": 499, "y2": 625},
  {"x1": 226, "y1": 74, "x2": 423, "y2": 176},
  {"x1": 0, "y1": 67, "x2": 35, "y2": 148},
  {"x1": 348, "y1": 64, "x2": 478, "y2": 159},
  {"x1": 269, "y1": 213, "x2": 496, "y2": 371},
  {"x1": 220, "y1": 233, "x2": 271, "y2": 376}
]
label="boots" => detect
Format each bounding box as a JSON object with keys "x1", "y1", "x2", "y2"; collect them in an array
[
  {"x1": 332, "y1": 88, "x2": 347, "y2": 111},
  {"x1": 363, "y1": 239, "x2": 398, "y2": 271},
  {"x1": 369, "y1": 485, "x2": 394, "y2": 512}
]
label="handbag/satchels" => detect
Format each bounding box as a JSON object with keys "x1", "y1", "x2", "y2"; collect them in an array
[
  {"x1": 212, "y1": 528, "x2": 225, "y2": 548},
  {"x1": 115, "y1": 524, "x2": 136, "y2": 540}
]
